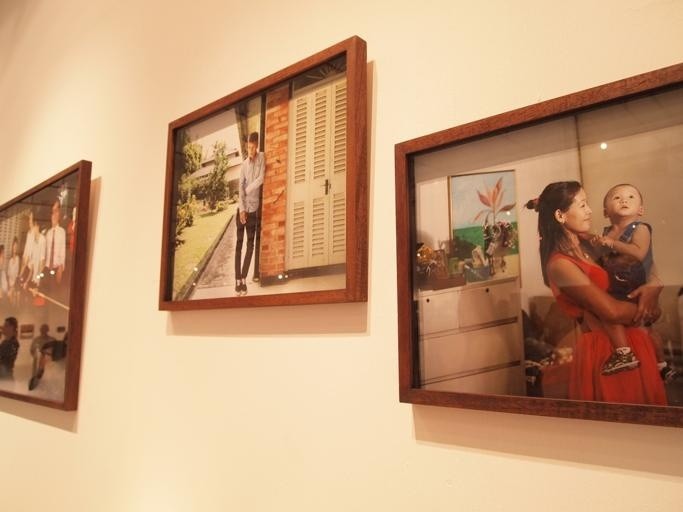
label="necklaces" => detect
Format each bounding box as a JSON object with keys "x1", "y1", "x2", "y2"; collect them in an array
[{"x1": 583, "y1": 251, "x2": 589, "y2": 260}]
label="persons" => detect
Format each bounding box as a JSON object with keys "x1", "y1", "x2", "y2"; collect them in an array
[
  {"x1": 0, "y1": 200, "x2": 69, "y2": 390},
  {"x1": 588, "y1": 184, "x2": 680, "y2": 386},
  {"x1": 232, "y1": 130, "x2": 265, "y2": 299},
  {"x1": 523, "y1": 179, "x2": 668, "y2": 408}
]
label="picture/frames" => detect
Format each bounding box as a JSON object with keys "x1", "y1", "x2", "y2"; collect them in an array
[
  {"x1": 0, "y1": 159, "x2": 91, "y2": 412},
  {"x1": 158, "y1": 35, "x2": 371, "y2": 309},
  {"x1": 392, "y1": 58, "x2": 683, "y2": 428}
]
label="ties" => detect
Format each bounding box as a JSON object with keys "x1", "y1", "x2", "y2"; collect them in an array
[{"x1": 49, "y1": 229, "x2": 57, "y2": 274}]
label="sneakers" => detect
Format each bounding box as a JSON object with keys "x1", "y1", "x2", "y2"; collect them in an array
[
  {"x1": 597, "y1": 346, "x2": 641, "y2": 378},
  {"x1": 658, "y1": 366, "x2": 679, "y2": 386},
  {"x1": 234, "y1": 277, "x2": 248, "y2": 296}
]
